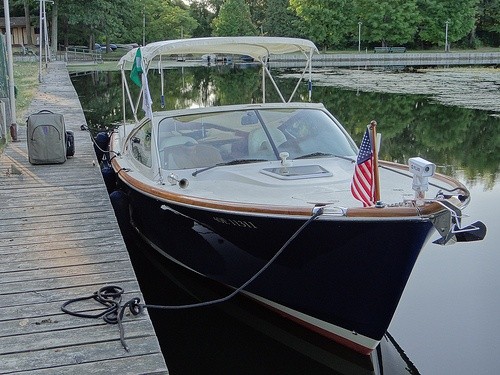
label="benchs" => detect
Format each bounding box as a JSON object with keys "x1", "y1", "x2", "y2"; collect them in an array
[{"x1": 374, "y1": 47, "x2": 407, "y2": 54}]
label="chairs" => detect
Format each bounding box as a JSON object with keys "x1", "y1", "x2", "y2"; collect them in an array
[
  {"x1": 248, "y1": 126, "x2": 288, "y2": 160},
  {"x1": 164, "y1": 143, "x2": 223, "y2": 170}
]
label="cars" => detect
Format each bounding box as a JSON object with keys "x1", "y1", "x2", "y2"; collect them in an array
[{"x1": 93, "y1": 47, "x2": 112, "y2": 53}]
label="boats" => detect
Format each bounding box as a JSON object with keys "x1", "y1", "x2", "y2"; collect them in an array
[{"x1": 91, "y1": 36, "x2": 488, "y2": 356}]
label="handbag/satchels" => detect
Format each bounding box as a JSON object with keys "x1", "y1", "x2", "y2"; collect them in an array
[
  {"x1": 64, "y1": 130, "x2": 75, "y2": 157},
  {"x1": 26, "y1": 109, "x2": 67, "y2": 165}
]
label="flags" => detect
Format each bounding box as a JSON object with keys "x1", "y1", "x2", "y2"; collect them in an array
[
  {"x1": 349, "y1": 125, "x2": 377, "y2": 208},
  {"x1": 129, "y1": 49, "x2": 144, "y2": 89}
]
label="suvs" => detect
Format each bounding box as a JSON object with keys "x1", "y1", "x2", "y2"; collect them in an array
[{"x1": 98, "y1": 44, "x2": 117, "y2": 50}]
